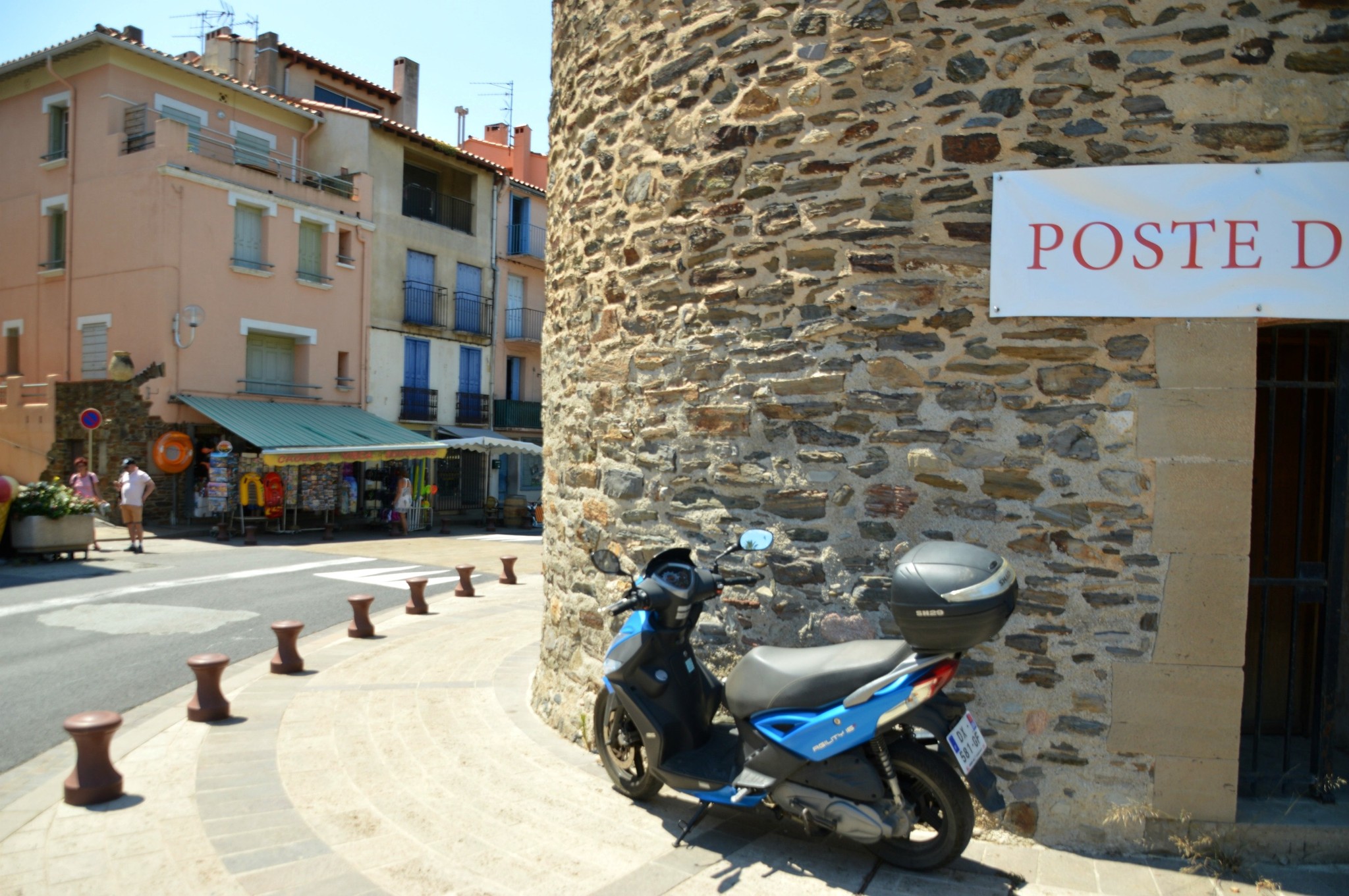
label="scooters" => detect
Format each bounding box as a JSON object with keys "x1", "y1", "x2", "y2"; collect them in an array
[{"x1": 590, "y1": 528, "x2": 1018, "y2": 874}]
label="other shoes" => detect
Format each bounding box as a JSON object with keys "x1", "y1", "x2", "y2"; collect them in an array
[
  {"x1": 135, "y1": 546, "x2": 143, "y2": 553},
  {"x1": 124, "y1": 546, "x2": 135, "y2": 551},
  {"x1": 401, "y1": 532, "x2": 408, "y2": 536},
  {"x1": 94, "y1": 545, "x2": 100, "y2": 551}
]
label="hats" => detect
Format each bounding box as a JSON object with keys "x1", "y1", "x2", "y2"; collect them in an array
[{"x1": 121, "y1": 457, "x2": 136, "y2": 466}]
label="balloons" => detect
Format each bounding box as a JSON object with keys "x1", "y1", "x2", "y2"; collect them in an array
[
  {"x1": 420, "y1": 484, "x2": 426, "y2": 495},
  {"x1": 422, "y1": 500, "x2": 430, "y2": 508},
  {"x1": 431, "y1": 485, "x2": 438, "y2": 495},
  {"x1": 426, "y1": 484, "x2": 431, "y2": 495}
]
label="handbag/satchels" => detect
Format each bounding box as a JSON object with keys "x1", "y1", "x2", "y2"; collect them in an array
[{"x1": 381, "y1": 505, "x2": 400, "y2": 522}]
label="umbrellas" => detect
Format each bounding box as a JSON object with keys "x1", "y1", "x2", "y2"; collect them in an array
[{"x1": 439, "y1": 434, "x2": 544, "y2": 505}]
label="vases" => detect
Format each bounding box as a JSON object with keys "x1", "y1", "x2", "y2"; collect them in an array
[{"x1": 11, "y1": 515, "x2": 95, "y2": 547}]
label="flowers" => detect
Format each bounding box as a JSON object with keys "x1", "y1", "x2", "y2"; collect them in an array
[{"x1": 9, "y1": 475, "x2": 100, "y2": 520}]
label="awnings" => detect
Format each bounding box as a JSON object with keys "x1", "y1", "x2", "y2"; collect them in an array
[
  {"x1": 436, "y1": 420, "x2": 509, "y2": 442},
  {"x1": 168, "y1": 392, "x2": 449, "y2": 467}
]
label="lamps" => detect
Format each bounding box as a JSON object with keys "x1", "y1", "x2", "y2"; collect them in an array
[{"x1": 172, "y1": 304, "x2": 206, "y2": 349}]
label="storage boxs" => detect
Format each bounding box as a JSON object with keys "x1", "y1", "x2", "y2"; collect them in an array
[
  {"x1": 193, "y1": 492, "x2": 213, "y2": 517},
  {"x1": 891, "y1": 540, "x2": 1019, "y2": 648}
]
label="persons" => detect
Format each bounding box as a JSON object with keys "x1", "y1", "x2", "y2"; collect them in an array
[
  {"x1": 69, "y1": 457, "x2": 105, "y2": 551},
  {"x1": 391, "y1": 465, "x2": 414, "y2": 536},
  {"x1": 112, "y1": 457, "x2": 156, "y2": 554}
]
